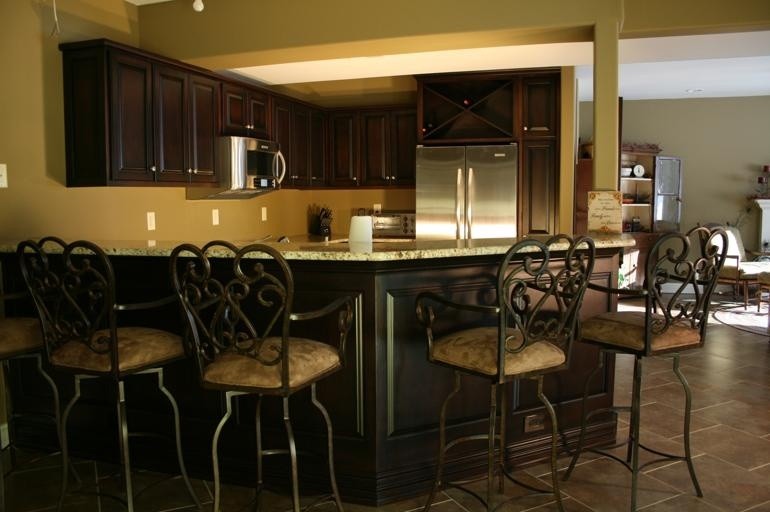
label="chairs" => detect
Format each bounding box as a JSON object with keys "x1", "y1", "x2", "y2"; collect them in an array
[
  {"x1": 0, "y1": 319, "x2": 64, "y2": 512},
  {"x1": 18, "y1": 237, "x2": 196, "y2": 512},
  {"x1": 414, "y1": 235, "x2": 595, "y2": 512},
  {"x1": 562, "y1": 230, "x2": 729, "y2": 512},
  {"x1": 170, "y1": 240, "x2": 355, "y2": 512}
]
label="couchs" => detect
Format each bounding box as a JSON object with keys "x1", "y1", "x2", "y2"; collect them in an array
[
  {"x1": 757, "y1": 268, "x2": 770, "y2": 312},
  {"x1": 697, "y1": 222, "x2": 770, "y2": 310}
]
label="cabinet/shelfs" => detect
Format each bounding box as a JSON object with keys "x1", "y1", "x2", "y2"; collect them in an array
[
  {"x1": 573, "y1": 143, "x2": 681, "y2": 236},
  {"x1": 59, "y1": 39, "x2": 155, "y2": 188},
  {"x1": 361, "y1": 103, "x2": 416, "y2": 189},
  {"x1": 516, "y1": 67, "x2": 561, "y2": 244},
  {"x1": 617, "y1": 233, "x2": 660, "y2": 299},
  {"x1": 155, "y1": 52, "x2": 222, "y2": 182},
  {"x1": 326, "y1": 108, "x2": 362, "y2": 189},
  {"x1": 273, "y1": 92, "x2": 325, "y2": 189},
  {"x1": 222, "y1": 76, "x2": 273, "y2": 140}
]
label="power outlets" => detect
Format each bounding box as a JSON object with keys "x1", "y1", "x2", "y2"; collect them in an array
[
  {"x1": 0, "y1": 164, "x2": 8, "y2": 188},
  {"x1": 373, "y1": 204, "x2": 382, "y2": 215}
]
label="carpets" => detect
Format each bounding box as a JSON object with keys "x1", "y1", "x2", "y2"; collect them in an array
[{"x1": 713, "y1": 293, "x2": 770, "y2": 337}]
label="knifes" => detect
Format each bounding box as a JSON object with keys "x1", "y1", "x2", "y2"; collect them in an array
[{"x1": 319, "y1": 206, "x2": 333, "y2": 222}]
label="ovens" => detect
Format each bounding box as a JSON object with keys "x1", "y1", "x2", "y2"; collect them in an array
[{"x1": 368, "y1": 209, "x2": 416, "y2": 239}]
